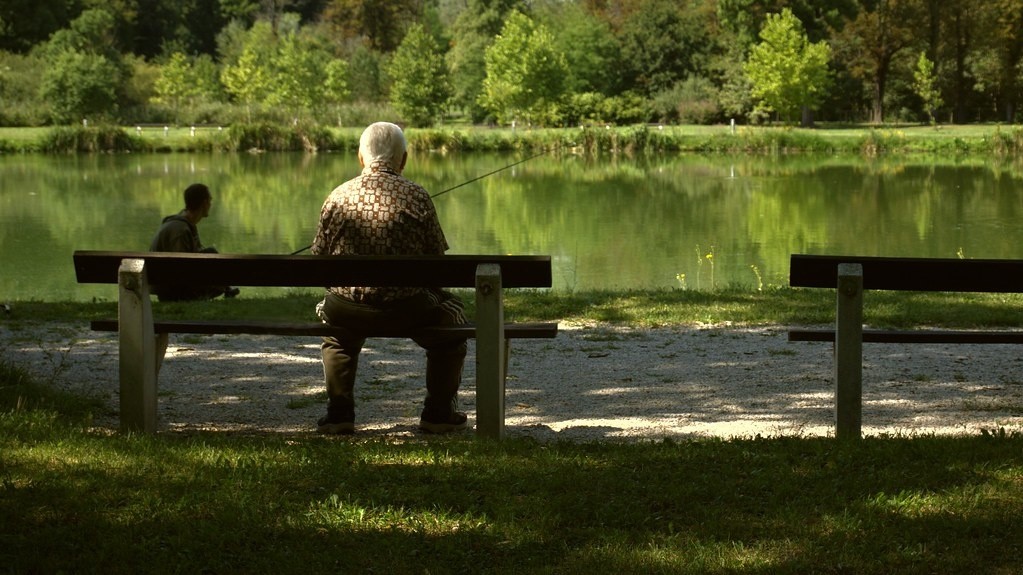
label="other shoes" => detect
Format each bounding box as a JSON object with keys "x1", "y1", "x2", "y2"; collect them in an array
[
  {"x1": 419, "y1": 404, "x2": 468, "y2": 431},
  {"x1": 316, "y1": 416, "x2": 354, "y2": 435},
  {"x1": 224, "y1": 287, "x2": 240, "y2": 299}
]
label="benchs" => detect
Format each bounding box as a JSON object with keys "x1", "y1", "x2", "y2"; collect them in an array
[
  {"x1": 788, "y1": 253, "x2": 1023, "y2": 440},
  {"x1": 71, "y1": 250, "x2": 558, "y2": 441}
]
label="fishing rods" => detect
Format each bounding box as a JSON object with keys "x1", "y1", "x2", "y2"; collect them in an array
[{"x1": 289, "y1": 131, "x2": 599, "y2": 256}]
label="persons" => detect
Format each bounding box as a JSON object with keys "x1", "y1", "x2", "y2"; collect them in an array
[
  {"x1": 148, "y1": 183, "x2": 236, "y2": 302},
  {"x1": 312, "y1": 122, "x2": 471, "y2": 433}
]
